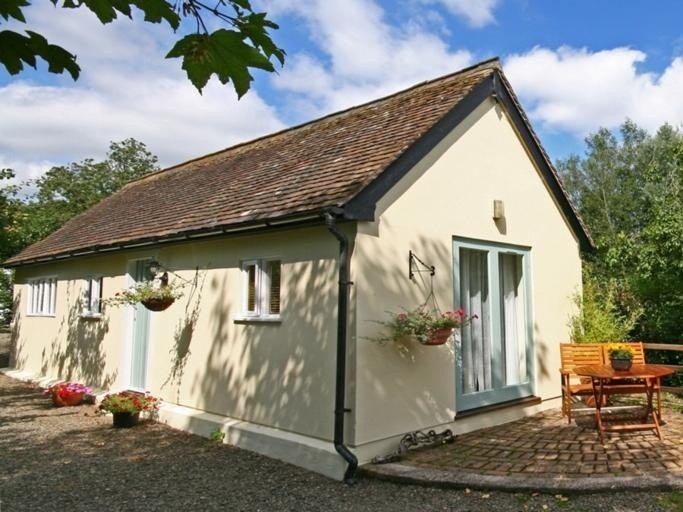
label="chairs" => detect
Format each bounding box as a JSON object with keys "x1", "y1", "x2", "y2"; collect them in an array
[{"x1": 557, "y1": 341, "x2": 677, "y2": 423}]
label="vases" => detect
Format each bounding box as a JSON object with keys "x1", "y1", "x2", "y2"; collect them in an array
[
  {"x1": 424, "y1": 328, "x2": 453, "y2": 346},
  {"x1": 114, "y1": 412, "x2": 141, "y2": 428},
  {"x1": 51, "y1": 393, "x2": 84, "y2": 406}
]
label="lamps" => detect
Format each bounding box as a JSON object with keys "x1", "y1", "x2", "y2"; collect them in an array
[
  {"x1": 148, "y1": 261, "x2": 158, "y2": 276},
  {"x1": 492, "y1": 197, "x2": 506, "y2": 220}
]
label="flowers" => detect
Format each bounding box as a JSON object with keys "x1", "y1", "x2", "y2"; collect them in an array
[
  {"x1": 92, "y1": 389, "x2": 163, "y2": 418},
  {"x1": 43, "y1": 382, "x2": 94, "y2": 398},
  {"x1": 358, "y1": 306, "x2": 479, "y2": 361}
]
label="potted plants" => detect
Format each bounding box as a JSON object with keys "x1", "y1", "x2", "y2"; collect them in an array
[{"x1": 98, "y1": 270, "x2": 193, "y2": 314}]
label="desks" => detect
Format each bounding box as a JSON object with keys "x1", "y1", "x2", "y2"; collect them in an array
[{"x1": 571, "y1": 363, "x2": 676, "y2": 444}]
